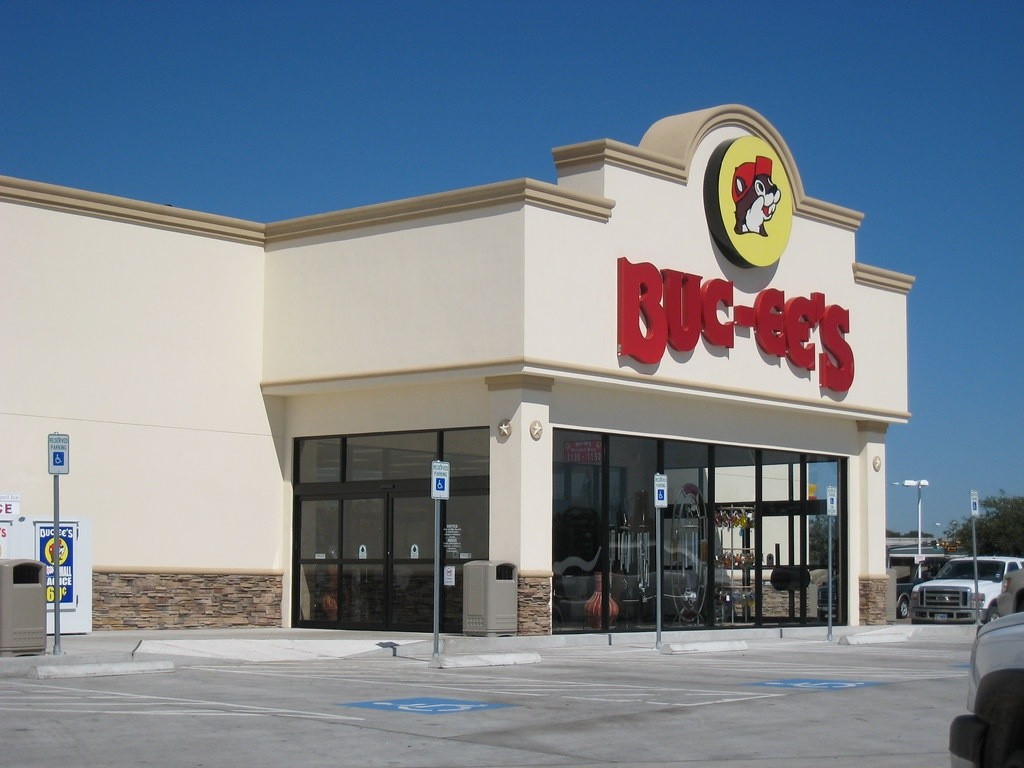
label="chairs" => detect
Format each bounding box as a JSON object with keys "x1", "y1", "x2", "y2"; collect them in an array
[{"x1": 553, "y1": 570, "x2": 708, "y2": 631}]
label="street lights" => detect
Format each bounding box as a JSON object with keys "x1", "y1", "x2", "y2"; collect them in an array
[
  {"x1": 935, "y1": 522, "x2": 957, "y2": 546},
  {"x1": 902, "y1": 477, "x2": 929, "y2": 582}
]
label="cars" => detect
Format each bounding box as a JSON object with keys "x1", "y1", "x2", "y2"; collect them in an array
[
  {"x1": 551, "y1": 539, "x2": 723, "y2": 622},
  {"x1": 995, "y1": 568, "x2": 1024, "y2": 617},
  {"x1": 940, "y1": 607, "x2": 1024, "y2": 768}
]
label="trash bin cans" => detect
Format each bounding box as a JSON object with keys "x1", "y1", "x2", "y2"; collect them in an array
[
  {"x1": 462, "y1": 560, "x2": 518, "y2": 638},
  {"x1": 0, "y1": 558, "x2": 47, "y2": 657}
]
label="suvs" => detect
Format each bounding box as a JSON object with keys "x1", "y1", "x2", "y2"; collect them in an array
[
  {"x1": 907, "y1": 553, "x2": 1024, "y2": 627},
  {"x1": 816, "y1": 555, "x2": 953, "y2": 623}
]
